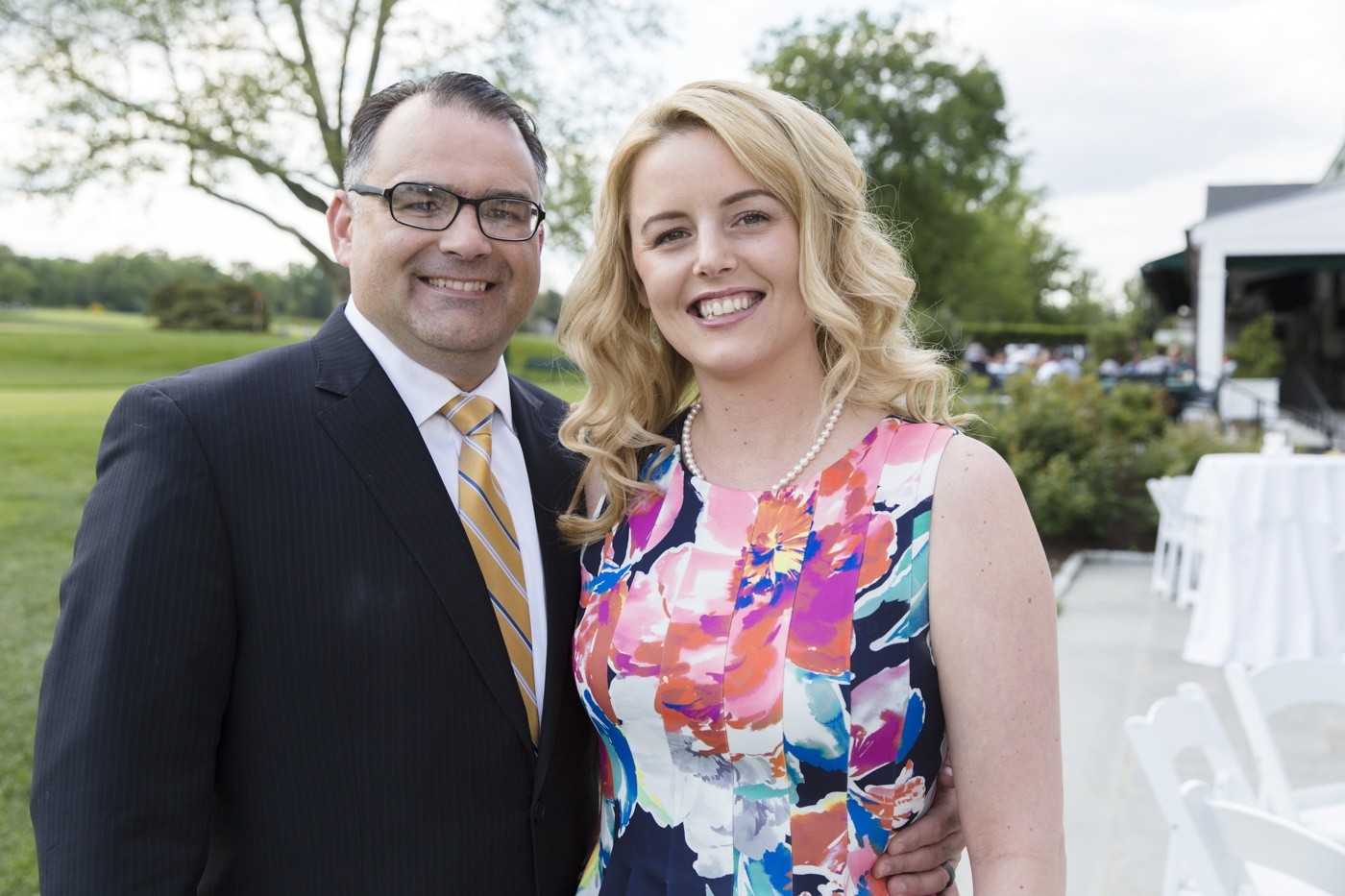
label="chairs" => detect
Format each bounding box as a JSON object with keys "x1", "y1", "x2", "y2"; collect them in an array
[{"x1": 1126, "y1": 440, "x2": 1345, "y2": 896}]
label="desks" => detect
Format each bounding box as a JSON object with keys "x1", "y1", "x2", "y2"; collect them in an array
[{"x1": 1198, "y1": 453, "x2": 1345, "y2": 673}]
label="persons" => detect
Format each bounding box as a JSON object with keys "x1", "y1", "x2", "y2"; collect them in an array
[
  {"x1": 564, "y1": 82, "x2": 1065, "y2": 896},
  {"x1": 960, "y1": 333, "x2": 1238, "y2": 393},
  {"x1": 30, "y1": 73, "x2": 969, "y2": 896},
  {"x1": 1265, "y1": 333, "x2": 1332, "y2": 408}
]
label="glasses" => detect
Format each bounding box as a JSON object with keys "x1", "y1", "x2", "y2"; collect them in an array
[{"x1": 349, "y1": 181, "x2": 546, "y2": 242}]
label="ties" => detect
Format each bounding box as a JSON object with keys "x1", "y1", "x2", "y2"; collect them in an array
[{"x1": 440, "y1": 393, "x2": 538, "y2": 743}]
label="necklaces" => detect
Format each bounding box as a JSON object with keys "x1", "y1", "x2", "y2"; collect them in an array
[{"x1": 682, "y1": 393, "x2": 845, "y2": 495}]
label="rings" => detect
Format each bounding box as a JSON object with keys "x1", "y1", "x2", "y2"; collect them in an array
[{"x1": 940, "y1": 862, "x2": 955, "y2": 888}]
